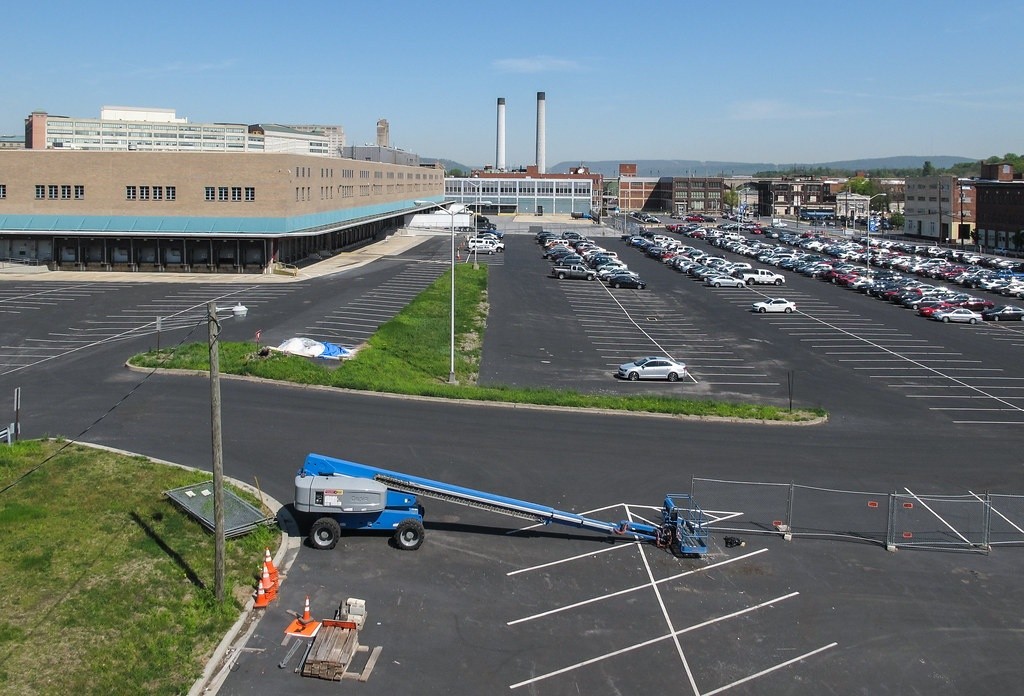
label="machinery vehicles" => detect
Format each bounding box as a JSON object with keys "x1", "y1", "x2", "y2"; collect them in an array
[{"x1": 293, "y1": 453, "x2": 710, "y2": 551}]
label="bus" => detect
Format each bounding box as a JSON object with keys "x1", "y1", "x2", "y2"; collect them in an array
[{"x1": 798, "y1": 208, "x2": 836, "y2": 222}]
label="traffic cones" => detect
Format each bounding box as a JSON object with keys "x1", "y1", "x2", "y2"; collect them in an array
[
  {"x1": 298, "y1": 595, "x2": 314, "y2": 624},
  {"x1": 259, "y1": 561, "x2": 274, "y2": 590},
  {"x1": 264, "y1": 546, "x2": 276, "y2": 574},
  {"x1": 253, "y1": 580, "x2": 268, "y2": 607}
]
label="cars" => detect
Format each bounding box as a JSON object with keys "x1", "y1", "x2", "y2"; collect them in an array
[
  {"x1": 618, "y1": 355, "x2": 688, "y2": 382},
  {"x1": 535, "y1": 210, "x2": 1024, "y2": 325},
  {"x1": 466, "y1": 224, "x2": 505, "y2": 255},
  {"x1": 752, "y1": 297, "x2": 797, "y2": 314}
]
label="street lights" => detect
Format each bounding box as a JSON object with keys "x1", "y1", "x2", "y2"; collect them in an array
[
  {"x1": 851, "y1": 193, "x2": 887, "y2": 281},
  {"x1": 735, "y1": 187, "x2": 751, "y2": 242},
  {"x1": 414, "y1": 200, "x2": 492, "y2": 384}
]
